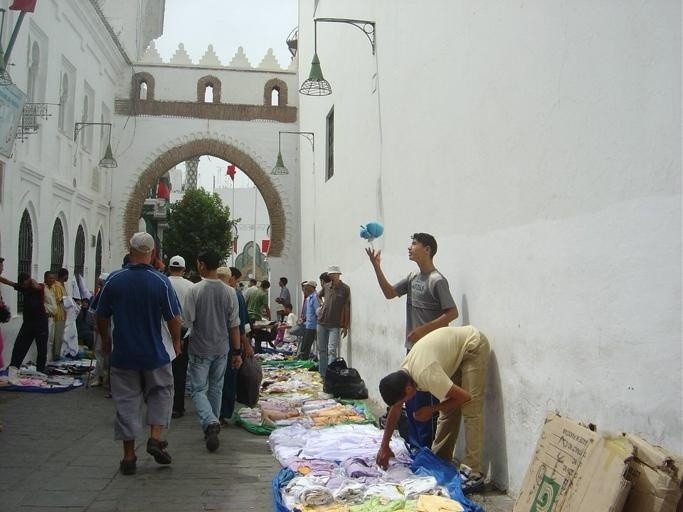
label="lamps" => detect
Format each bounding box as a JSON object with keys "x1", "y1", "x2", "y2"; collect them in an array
[
  {"x1": 73, "y1": 121, "x2": 118, "y2": 168},
  {"x1": 300, "y1": 18, "x2": 376, "y2": 95},
  {"x1": 1, "y1": 13, "x2": 13, "y2": 85},
  {"x1": 270, "y1": 131, "x2": 316, "y2": 175}
]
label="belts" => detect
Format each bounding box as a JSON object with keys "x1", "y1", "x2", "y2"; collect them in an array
[
  {"x1": 24, "y1": 318, "x2": 48, "y2": 322},
  {"x1": 48, "y1": 315, "x2": 55, "y2": 317}
]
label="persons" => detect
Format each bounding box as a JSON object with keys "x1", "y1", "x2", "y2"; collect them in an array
[
  {"x1": 53, "y1": 268, "x2": 70, "y2": 363},
  {"x1": 364, "y1": 232, "x2": 459, "y2": 460},
  {"x1": 76, "y1": 297, "x2": 94, "y2": 349},
  {"x1": 318, "y1": 272, "x2": 331, "y2": 309},
  {"x1": 93, "y1": 232, "x2": 184, "y2": 474},
  {"x1": 41, "y1": 270, "x2": 57, "y2": 364},
  {"x1": 275, "y1": 277, "x2": 290, "y2": 309},
  {"x1": 0, "y1": 257, "x2": 11, "y2": 369},
  {"x1": 182, "y1": 248, "x2": 242, "y2": 452},
  {"x1": 104, "y1": 253, "x2": 130, "y2": 284},
  {"x1": 88, "y1": 272, "x2": 113, "y2": 399},
  {"x1": 166, "y1": 255, "x2": 196, "y2": 419},
  {"x1": 228, "y1": 266, "x2": 251, "y2": 335},
  {"x1": 375, "y1": 326, "x2": 491, "y2": 494},
  {"x1": 314, "y1": 265, "x2": 350, "y2": 383},
  {"x1": 290, "y1": 281, "x2": 309, "y2": 338},
  {"x1": 0, "y1": 271, "x2": 48, "y2": 375},
  {"x1": 297, "y1": 280, "x2": 321, "y2": 361},
  {"x1": 243, "y1": 278, "x2": 258, "y2": 306},
  {"x1": 215, "y1": 266, "x2": 238, "y2": 430},
  {"x1": 276, "y1": 302, "x2": 297, "y2": 345},
  {"x1": 246, "y1": 280, "x2": 271, "y2": 354}
]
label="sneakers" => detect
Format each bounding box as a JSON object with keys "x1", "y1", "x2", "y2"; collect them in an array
[
  {"x1": 459, "y1": 464, "x2": 485, "y2": 496},
  {"x1": 147, "y1": 438, "x2": 172, "y2": 464},
  {"x1": 172, "y1": 411, "x2": 184, "y2": 418},
  {"x1": 120, "y1": 457, "x2": 138, "y2": 475},
  {"x1": 91, "y1": 377, "x2": 103, "y2": 387},
  {"x1": 319, "y1": 377, "x2": 326, "y2": 382},
  {"x1": 205, "y1": 421, "x2": 220, "y2": 451},
  {"x1": 218, "y1": 419, "x2": 228, "y2": 428}
]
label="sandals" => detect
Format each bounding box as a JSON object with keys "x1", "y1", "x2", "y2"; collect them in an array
[{"x1": 105, "y1": 392, "x2": 113, "y2": 398}]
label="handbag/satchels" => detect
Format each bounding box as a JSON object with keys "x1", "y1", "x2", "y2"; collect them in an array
[
  {"x1": 0, "y1": 301, "x2": 11, "y2": 323},
  {"x1": 236, "y1": 348, "x2": 262, "y2": 405}
]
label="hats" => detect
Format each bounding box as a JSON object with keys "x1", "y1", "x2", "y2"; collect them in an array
[
  {"x1": 303, "y1": 280, "x2": 317, "y2": 288},
  {"x1": 216, "y1": 267, "x2": 231, "y2": 276},
  {"x1": 328, "y1": 265, "x2": 342, "y2": 275},
  {"x1": 99, "y1": 273, "x2": 109, "y2": 280},
  {"x1": 130, "y1": 232, "x2": 154, "y2": 253},
  {"x1": 18, "y1": 272, "x2": 30, "y2": 285},
  {"x1": 169, "y1": 255, "x2": 185, "y2": 267}
]
